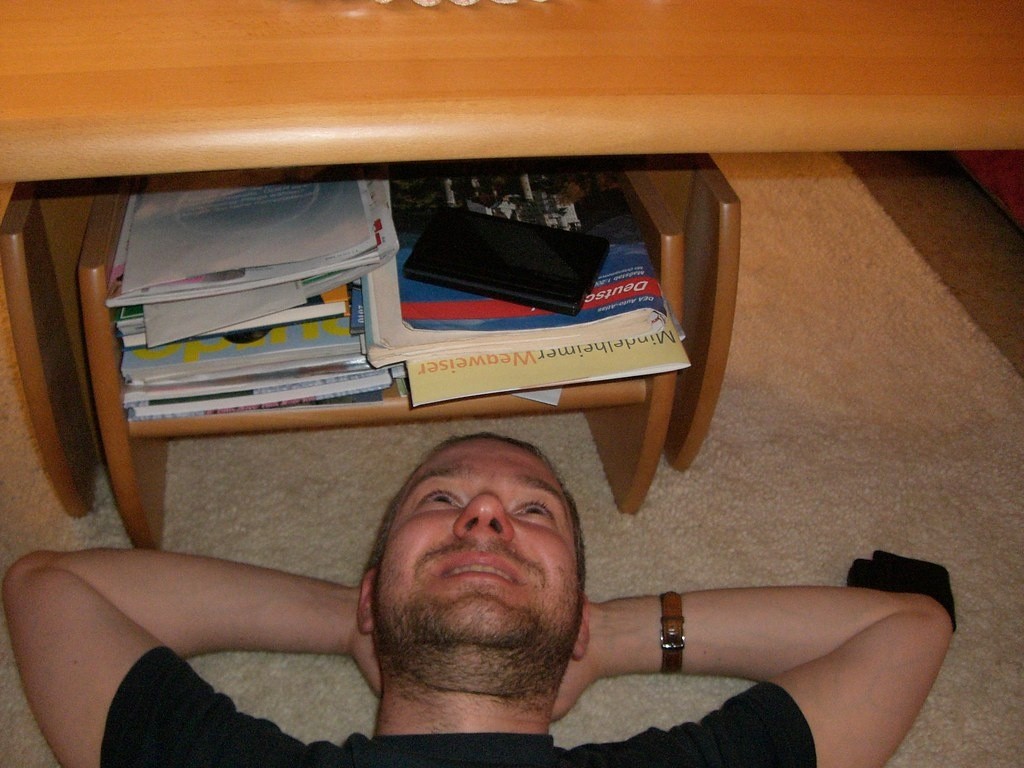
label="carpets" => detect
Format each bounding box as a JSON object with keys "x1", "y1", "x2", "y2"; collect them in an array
[{"x1": 1, "y1": 152, "x2": 1022, "y2": 768}]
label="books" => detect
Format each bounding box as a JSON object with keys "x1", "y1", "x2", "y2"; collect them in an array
[{"x1": 106, "y1": 171, "x2": 693, "y2": 421}]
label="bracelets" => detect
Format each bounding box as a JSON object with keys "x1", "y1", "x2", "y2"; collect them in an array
[{"x1": 660, "y1": 589, "x2": 686, "y2": 674}]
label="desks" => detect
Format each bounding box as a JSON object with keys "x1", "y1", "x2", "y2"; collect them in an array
[{"x1": 1, "y1": 0, "x2": 1024, "y2": 522}]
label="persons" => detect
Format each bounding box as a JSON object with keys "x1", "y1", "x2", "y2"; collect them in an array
[{"x1": 0, "y1": 432, "x2": 954, "y2": 768}]
